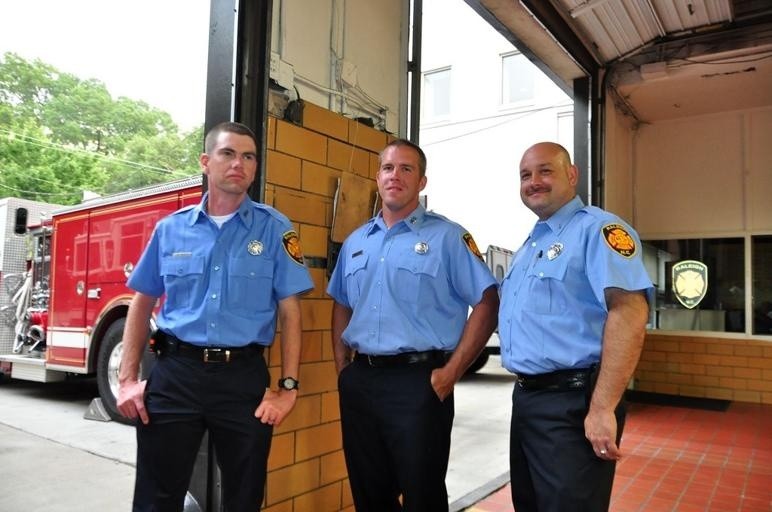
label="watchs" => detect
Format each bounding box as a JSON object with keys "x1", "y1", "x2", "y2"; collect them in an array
[{"x1": 278, "y1": 376, "x2": 299, "y2": 390}]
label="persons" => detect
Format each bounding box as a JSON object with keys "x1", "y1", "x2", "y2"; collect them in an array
[
  {"x1": 497, "y1": 141, "x2": 657, "y2": 512},
  {"x1": 324, "y1": 139, "x2": 501, "y2": 512},
  {"x1": 115, "y1": 121, "x2": 316, "y2": 512}
]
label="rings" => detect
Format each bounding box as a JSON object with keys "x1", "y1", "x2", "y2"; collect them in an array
[{"x1": 600, "y1": 449, "x2": 607, "y2": 455}]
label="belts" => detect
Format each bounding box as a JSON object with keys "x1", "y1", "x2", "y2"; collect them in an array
[
  {"x1": 353, "y1": 349, "x2": 446, "y2": 367},
  {"x1": 517, "y1": 365, "x2": 593, "y2": 391},
  {"x1": 169, "y1": 342, "x2": 264, "y2": 360}
]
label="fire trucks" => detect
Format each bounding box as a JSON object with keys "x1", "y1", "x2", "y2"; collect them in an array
[{"x1": 0, "y1": 173, "x2": 202, "y2": 427}]
